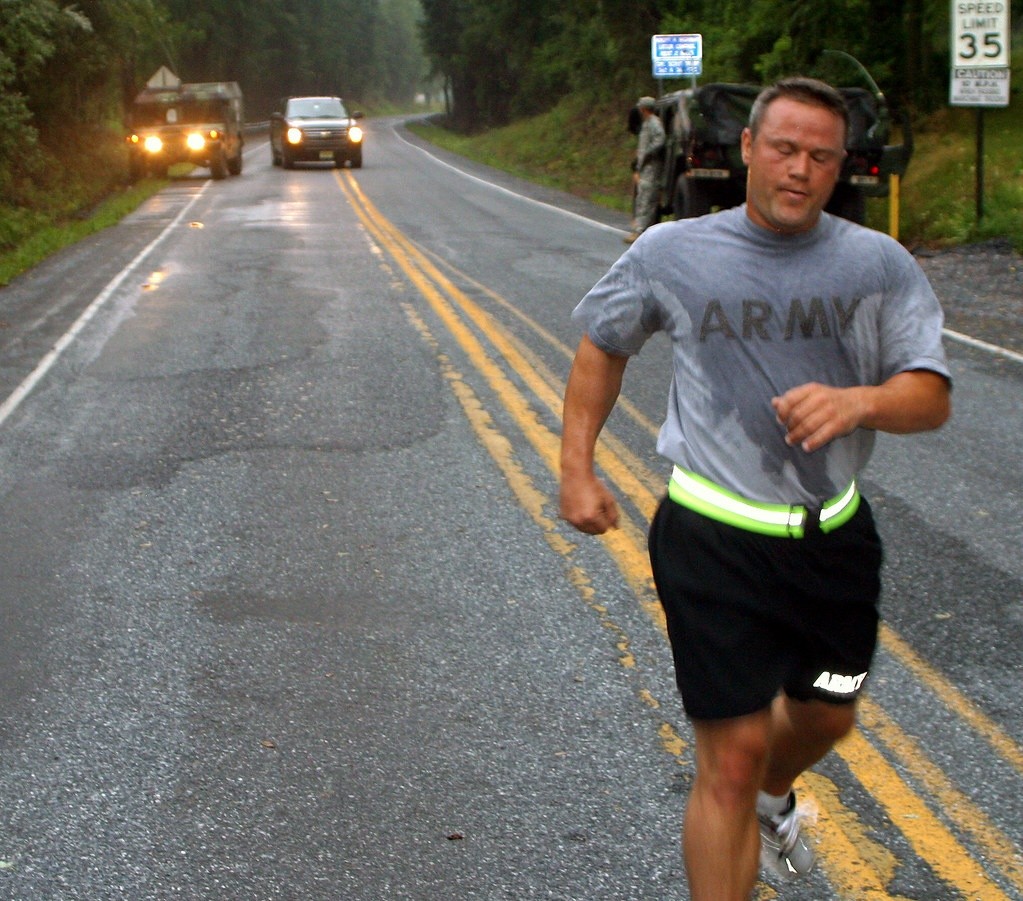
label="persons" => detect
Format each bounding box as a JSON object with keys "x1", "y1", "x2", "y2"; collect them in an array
[
  {"x1": 562, "y1": 75, "x2": 956, "y2": 901},
  {"x1": 625, "y1": 96, "x2": 665, "y2": 241}
]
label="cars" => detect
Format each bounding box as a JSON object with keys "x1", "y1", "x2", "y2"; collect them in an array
[{"x1": 269, "y1": 95, "x2": 364, "y2": 168}]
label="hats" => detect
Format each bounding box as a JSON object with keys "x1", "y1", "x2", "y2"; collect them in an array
[{"x1": 636, "y1": 97, "x2": 656, "y2": 109}]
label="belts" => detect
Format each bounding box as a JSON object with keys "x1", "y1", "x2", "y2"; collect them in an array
[{"x1": 669, "y1": 464, "x2": 859, "y2": 540}]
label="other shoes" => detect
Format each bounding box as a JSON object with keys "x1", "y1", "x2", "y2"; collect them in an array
[
  {"x1": 757, "y1": 787, "x2": 815, "y2": 880},
  {"x1": 625, "y1": 232, "x2": 640, "y2": 243}
]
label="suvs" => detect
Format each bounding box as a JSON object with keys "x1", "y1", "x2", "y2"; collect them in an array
[
  {"x1": 131, "y1": 80, "x2": 244, "y2": 181},
  {"x1": 630, "y1": 81, "x2": 870, "y2": 233}
]
